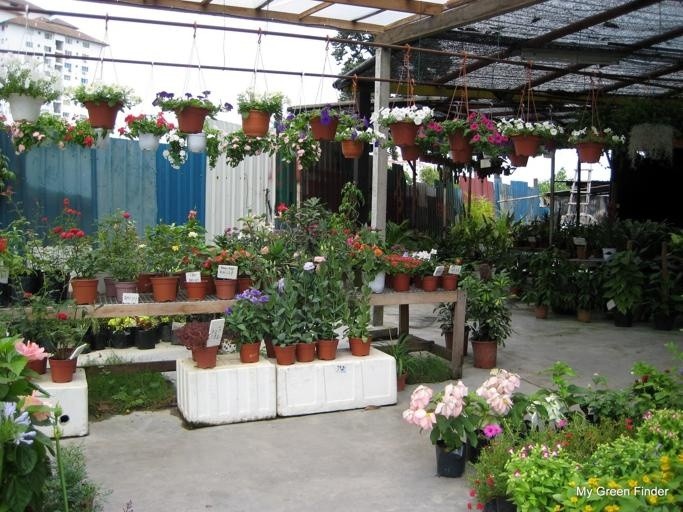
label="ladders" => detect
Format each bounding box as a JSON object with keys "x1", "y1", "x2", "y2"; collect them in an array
[{"x1": 565, "y1": 161, "x2": 592, "y2": 225}]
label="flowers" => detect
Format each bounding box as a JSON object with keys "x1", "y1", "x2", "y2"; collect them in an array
[{"x1": 0, "y1": 60, "x2": 683, "y2": 512}]
